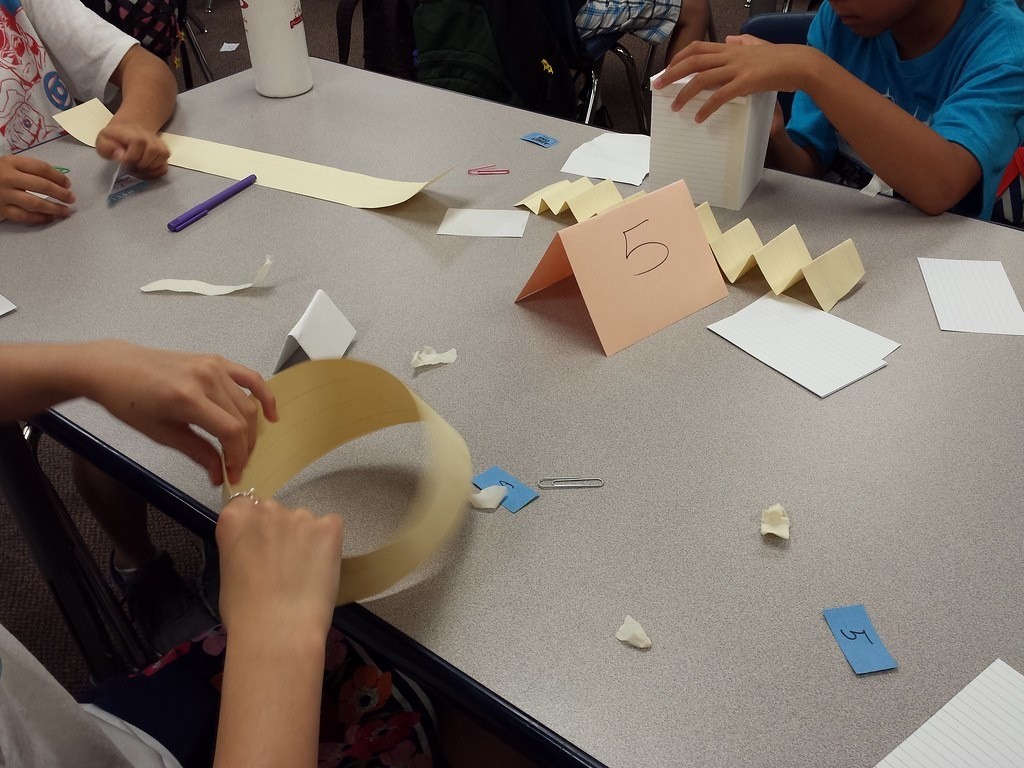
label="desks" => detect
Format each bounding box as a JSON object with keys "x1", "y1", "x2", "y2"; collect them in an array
[{"x1": 0, "y1": 56, "x2": 1023, "y2": 767}]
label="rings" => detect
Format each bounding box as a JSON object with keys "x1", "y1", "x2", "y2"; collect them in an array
[{"x1": 229, "y1": 487, "x2": 259, "y2": 507}]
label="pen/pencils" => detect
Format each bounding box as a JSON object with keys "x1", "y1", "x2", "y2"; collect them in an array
[{"x1": 166, "y1": 173, "x2": 258, "y2": 233}]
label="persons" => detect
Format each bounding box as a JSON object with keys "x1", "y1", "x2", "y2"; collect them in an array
[
  {"x1": 653, "y1": 0, "x2": 1024, "y2": 223},
  {"x1": 0, "y1": 340, "x2": 443, "y2": 768},
  {"x1": 569, "y1": 0, "x2": 711, "y2": 69},
  {"x1": 0, "y1": 0, "x2": 222, "y2": 653}
]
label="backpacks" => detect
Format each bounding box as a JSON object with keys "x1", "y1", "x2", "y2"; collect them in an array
[{"x1": 412, "y1": 0, "x2": 577, "y2": 123}]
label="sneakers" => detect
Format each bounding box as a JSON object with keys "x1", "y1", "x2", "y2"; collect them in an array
[
  {"x1": 187, "y1": 529, "x2": 223, "y2": 623},
  {"x1": 109, "y1": 541, "x2": 221, "y2": 659}
]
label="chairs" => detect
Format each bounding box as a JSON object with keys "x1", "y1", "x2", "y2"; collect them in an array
[{"x1": 561, "y1": 1, "x2": 649, "y2": 136}]
